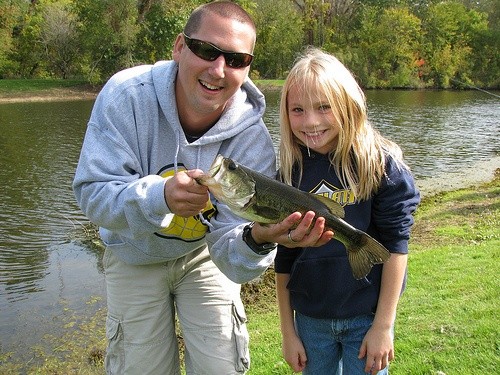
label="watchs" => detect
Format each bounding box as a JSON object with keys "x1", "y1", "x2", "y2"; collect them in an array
[{"x1": 242, "y1": 220, "x2": 278, "y2": 255}]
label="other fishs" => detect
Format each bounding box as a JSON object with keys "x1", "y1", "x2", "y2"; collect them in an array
[{"x1": 194, "y1": 153, "x2": 392, "y2": 280}]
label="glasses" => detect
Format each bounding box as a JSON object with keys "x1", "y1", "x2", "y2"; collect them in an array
[{"x1": 183, "y1": 33, "x2": 255, "y2": 69}]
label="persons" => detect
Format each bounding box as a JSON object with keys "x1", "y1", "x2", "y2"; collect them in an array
[
  {"x1": 274, "y1": 46, "x2": 421, "y2": 375},
  {"x1": 72, "y1": 1, "x2": 334, "y2": 375}
]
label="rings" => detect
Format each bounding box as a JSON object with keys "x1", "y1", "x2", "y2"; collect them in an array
[{"x1": 288, "y1": 231, "x2": 296, "y2": 244}]
